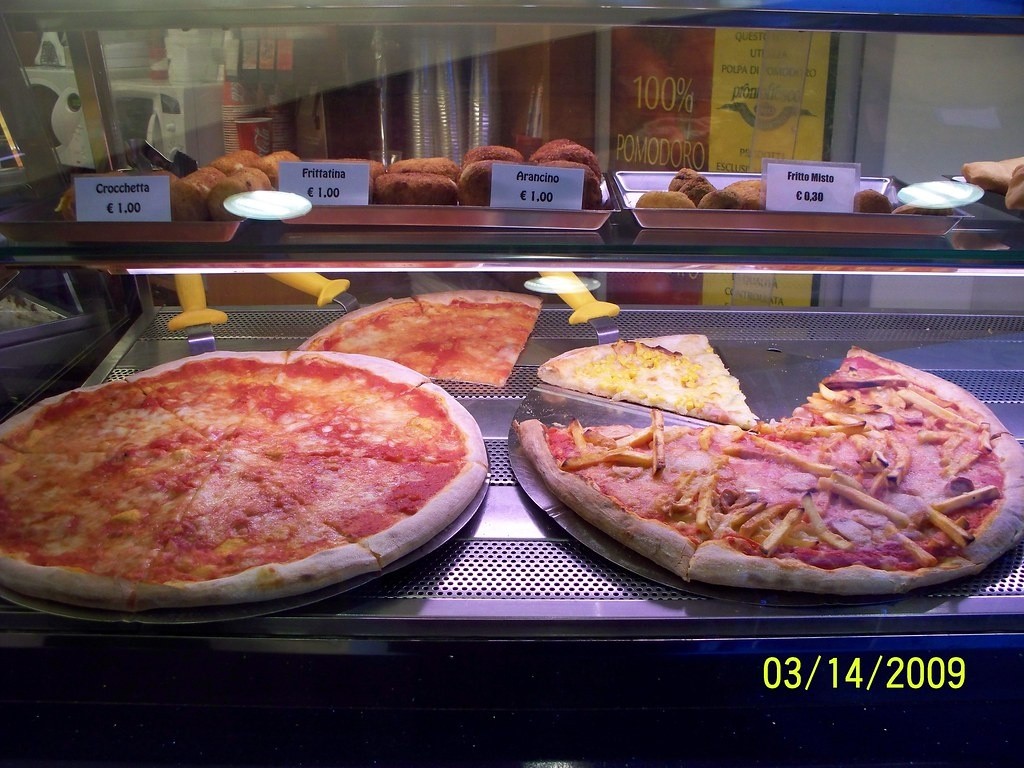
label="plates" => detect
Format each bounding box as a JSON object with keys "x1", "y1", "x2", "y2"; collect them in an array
[
  {"x1": 898, "y1": 181, "x2": 985, "y2": 209},
  {"x1": 222, "y1": 190, "x2": 312, "y2": 221}
]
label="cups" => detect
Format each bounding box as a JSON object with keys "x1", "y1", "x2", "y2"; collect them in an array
[
  {"x1": 468, "y1": 44, "x2": 499, "y2": 149},
  {"x1": 233, "y1": 116, "x2": 274, "y2": 155},
  {"x1": 411, "y1": 37, "x2": 439, "y2": 160},
  {"x1": 222, "y1": 76, "x2": 242, "y2": 158},
  {"x1": 434, "y1": 40, "x2": 465, "y2": 164},
  {"x1": 267, "y1": 84, "x2": 291, "y2": 152},
  {"x1": 371, "y1": 149, "x2": 400, "y2": 166}
]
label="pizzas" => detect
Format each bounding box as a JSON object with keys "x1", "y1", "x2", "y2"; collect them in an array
[
  {"x1": 535, "y1": 333, "x2": 760, "y2": 432},
  {"x1": 285, "y1": 289, "x2": 544, "y2": 388},
  {"x1": 0, "y1": 351, "x2": 489, "y2": 609}
]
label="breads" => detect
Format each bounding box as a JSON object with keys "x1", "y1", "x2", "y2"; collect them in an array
[
  {"x1": 57, "y1": 136, "x2": 604, "y2": 211},
  {"x1": 853, "y1": 190, "x2": 894, "y2": 213},
  {"x1": 637, "y1": 168, "x2": 762, "y2": 211}
]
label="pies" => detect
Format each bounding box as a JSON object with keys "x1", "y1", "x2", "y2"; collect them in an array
[{"x1": 510, "y1": 343, "x2": 1024, "y2": 593}]
